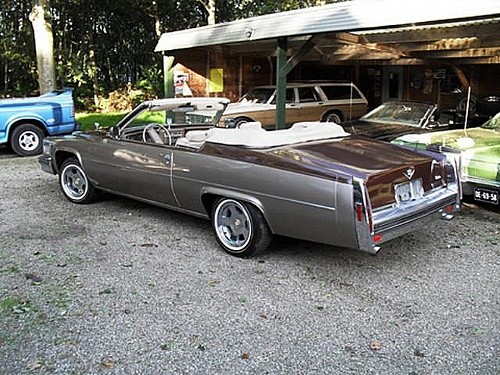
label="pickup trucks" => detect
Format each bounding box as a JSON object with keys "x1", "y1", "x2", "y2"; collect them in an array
[{"x1": 0, "y1": 86, "x2": 75, "y2": 157}]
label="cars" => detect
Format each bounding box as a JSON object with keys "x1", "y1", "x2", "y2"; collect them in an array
[
  {"x1": 190, "y1": 78, "x2": 367, "y2": 134},
  {"x1": 334, "y1": 92, "x2": 493, "y2": 143}
]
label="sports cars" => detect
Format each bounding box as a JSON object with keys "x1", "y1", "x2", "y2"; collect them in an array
[
  {"x1": 39, "y1": 96, "x2": 462, "y2": 260},
  {"x1": 391, "y1": 110, "x2": 500, "y2": 211}
]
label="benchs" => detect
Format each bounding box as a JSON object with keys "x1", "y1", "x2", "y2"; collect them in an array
[{"x1": 207, "y1": 120, "x2": 348, "y2": 152}]
